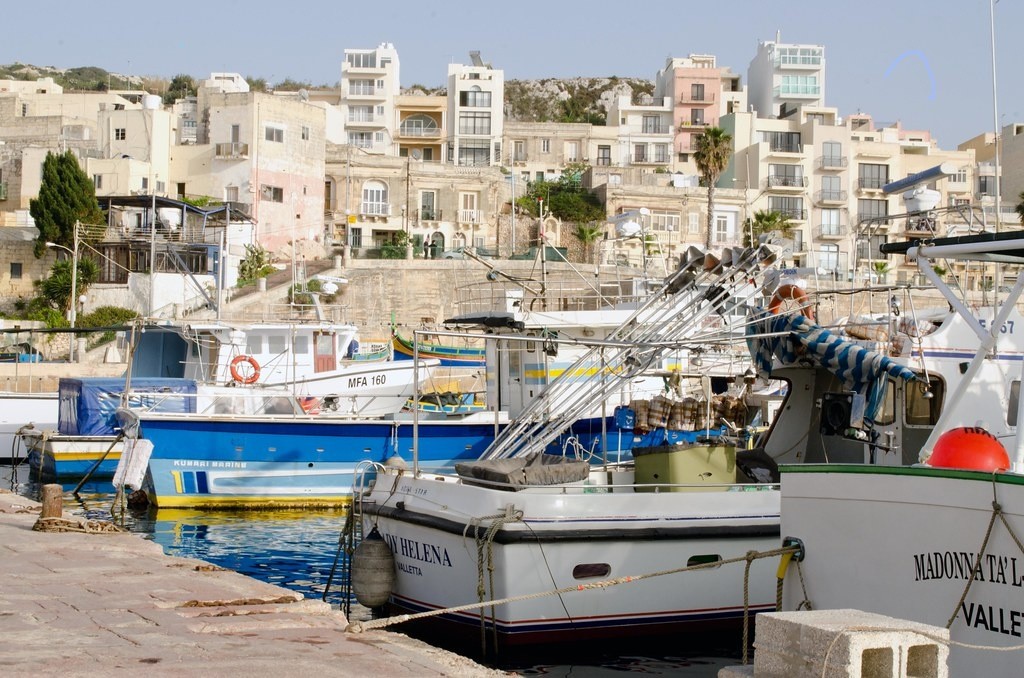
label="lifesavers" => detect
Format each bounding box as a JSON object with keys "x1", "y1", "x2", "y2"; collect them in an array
[
  {"x1": 230, "y1": 355, "x2": 261, "y2": 384},
  {"x1": 768, "y1": 284, "x2": 814, "y2": 325}
]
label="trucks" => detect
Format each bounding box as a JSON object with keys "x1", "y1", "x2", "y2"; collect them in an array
[{"x1": 510, "y1": 245, "x2": 568, "y2": 261}]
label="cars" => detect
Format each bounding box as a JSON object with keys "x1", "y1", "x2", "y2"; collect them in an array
[{"x1": 441, "y1": 244, "x2": 496, "y2": 260}]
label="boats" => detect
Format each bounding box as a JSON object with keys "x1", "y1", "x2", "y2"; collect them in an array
[{"x1": 1, "y1": 229, "x2": 1024, "y2": 677}]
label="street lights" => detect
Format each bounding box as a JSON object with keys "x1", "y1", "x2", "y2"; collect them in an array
[
  {"x1": 402, "y1": 204, "x2": 407, "y2": 229},
  {"x1": 471, "y1": 214, "x2": 476, "y2": 246},
  {"x1": 667, "y1": 226, "x2": 672, "y2": 260},
  {"x1": 346, "y1": 209, "x2": 350, "y2": 245},
  {"x1": 44, "y1": 243, "x2": 76, "y2": 363}
]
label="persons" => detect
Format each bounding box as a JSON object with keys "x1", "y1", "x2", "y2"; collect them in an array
[
  {"x1": 424, "y1": 238, "x2": 430, "y2": 260},
  {"x1": 430, "y1": 240, "x2": 437, "y2": 260}
]
